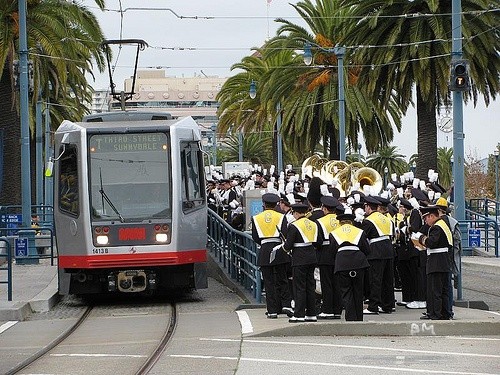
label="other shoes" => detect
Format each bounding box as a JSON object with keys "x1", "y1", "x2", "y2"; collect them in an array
[
  {"x1": 289, "y1": 316, "x2": 305, "y2": 322},
  {"x1": 305, "y1": 315, "x2": 317, "y2": 321},
  {"x1": 364, "y1": 299, "x2": 369, "y2": 304},
  {"x1": 363, "y1": 309, "x2": 378, "y2": 314},
  {"x1": 268, "y1": 312, "x2": 278, "y2": 318},
  {"x1": 335, "y1": 314, "x2": 341, "y2": 319},
  {"x1": 394, "y1": 288, "x2": 402, "y2": 290},
  {"x1": 419, "y1": 312, "x2": 432, "y2": 319},
  {"x1": 317, "y1": 312, "x2": 334, "y2": 318},
  {"x1": 406, "y1": 301, "x2": 427, "y2": 309},
  {"x1": 396, "y1": 302, "x2": 409, "y2": 306},
  {"x1": 378, "y1": 306, "x2": 396, "y2": 313},
  {"x1": 281, "y1": 307, "x2": 295, "y2": 317}
]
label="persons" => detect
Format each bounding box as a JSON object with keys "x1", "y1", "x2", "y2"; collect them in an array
[
  {"x1": 279, "y1": 205, "x2": 319, "y2": 322},
  {"x1": 249, "y1": 193, "x2": 296, "y2": 318},
  {"x1": 202, "y1": 160, "x2": 461, "y2": 314},
  {"x1": 416, "y1": 205, "x2": 456, "y2": 320},
  {"x1": 328, "y1": 215, "x2": 372, "y2": 321},
  {"x1": 313, "y1": 195, "x2": 343, "y2": 319}
]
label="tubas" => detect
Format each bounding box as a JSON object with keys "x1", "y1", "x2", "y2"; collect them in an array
[{"x1": 302, "y1": 155, "x2": 382, "y2": 196}]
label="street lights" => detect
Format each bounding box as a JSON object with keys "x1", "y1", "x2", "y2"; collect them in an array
[
  {"x1": 249, "y1": 79, "x2": 283, "y2": 175},
  {"x1": 303, "y1": 40, "x2": 347, "y2": 162}
]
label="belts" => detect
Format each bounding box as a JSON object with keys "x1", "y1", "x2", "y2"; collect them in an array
[
  {"x1": 260, "y1": 238, "x2": 281, "y2": 245},
  {"x1": 321, "y1": 241, "x2": 330, "y2": 245},
  {"x1": 426, "y1": 247, "x2": 448, "y2": 256},
  {"x1": 293, "y1": 242, "x2": 313, "y2": 247},
  {"x1": 369, "y1": 236, "x2": 390, "y2": 246},
  {"x1": 337, "y1": 245, "x2": 360, "y2": 252}
]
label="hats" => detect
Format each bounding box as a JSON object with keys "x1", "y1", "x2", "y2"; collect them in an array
[{"x1": 206, "y1": 164, "x2": 451, "y2": 221}]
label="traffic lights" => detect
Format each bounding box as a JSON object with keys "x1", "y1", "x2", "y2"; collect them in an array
[{"x1": 450, "y1": 58, "x2": 471, "y2": 92}]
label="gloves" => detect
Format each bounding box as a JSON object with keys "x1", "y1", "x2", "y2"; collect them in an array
[{"x1": 411, "y1": 232, "x2": 424, "y2": 241}]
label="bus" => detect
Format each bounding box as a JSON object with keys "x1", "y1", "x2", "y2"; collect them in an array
[{"x1": 53, "y1": 110, "x2": 208, "y2": 303}]
